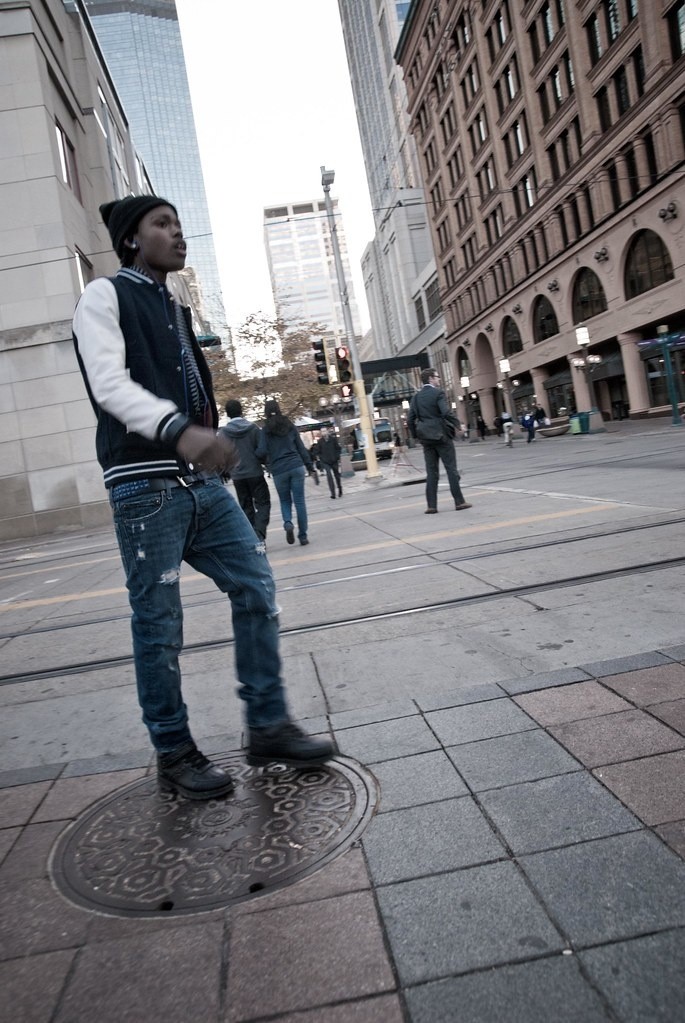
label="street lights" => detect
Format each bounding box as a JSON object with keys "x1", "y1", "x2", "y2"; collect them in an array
[
  {"x1": 457, "y1": 373, "x2": 482, "y2": 444},
  {"x1": 496, "y1": 356, "x2": 524, "y2": 439},
  {"x1": 319, "y1": 395, "x2": 355, "y2": 477},
  {"x1": 400, "y1": 399, "x2": 416, "y2": 448},
  {"x1": 571, "y1": 322, "x2": 608, "y2": 434},
  {"x1": 446, "y1": 386, "x2": 463, "y2": 442}
]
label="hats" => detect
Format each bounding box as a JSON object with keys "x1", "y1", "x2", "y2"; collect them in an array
[{"x1": 99, "y1": 194, "x2": 177, "y2": 258}]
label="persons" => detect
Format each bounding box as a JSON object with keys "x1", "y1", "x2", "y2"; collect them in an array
[
  {"x1": 493, "y1": 411, "x2": 513, "y2": 437},
  {"x1": 71, "y1": 194, "x2": 338, "y2": 801},
  {"x1": 394, "y1": 433, "x2": 400, "y2": 446},
  {"x1": 217, "y1": 399, "x2": 272, "y2": 542},
  {"x1": 461, "y1": 422, "x2": 469, "y2": 438},
  {"x1": 259, "y1": 400, "x2": 316, "y2": 545},
  {"x1": 311, "y1": 428, "x2": 342, "y2": 498},
  {"x1": 407, "y1": 368, "x2": 470, "y2": 513},
  {"x1": 521, "y1": 403, "x2": 546, "y2": 442},
  {"x1": 477, "y1": 416, "x2": 486, "y2": 440}
]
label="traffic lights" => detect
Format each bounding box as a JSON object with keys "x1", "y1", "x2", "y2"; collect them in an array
[
  {"x1": 341, "y1": 384, "x2": 354, "y2": 397},
  {"x1": 335, "y1": 346, "x2": 351, "y2": 383},
  {"x1": 312, "y1": 338, "x2": 332, "y2": 386}
]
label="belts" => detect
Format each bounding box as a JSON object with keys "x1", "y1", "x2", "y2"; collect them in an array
[{"x1": 112, "y1": 470, "x2": 220, "y2": 502}]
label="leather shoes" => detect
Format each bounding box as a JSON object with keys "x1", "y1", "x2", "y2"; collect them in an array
[
  {"x1": 246, "y1": 720, "x2": 336, "y2": 769},
  {"x1": 157, "y1": 743, "x2": 234, "y2": 800}
]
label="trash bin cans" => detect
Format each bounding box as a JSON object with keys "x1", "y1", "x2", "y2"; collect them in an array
[{"x1": 569, "y1": 412, "x2": 589, "y2": 435}]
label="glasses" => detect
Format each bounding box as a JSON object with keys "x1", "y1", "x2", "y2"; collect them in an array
[{"x1": 432, "y1": 376, "x2": 440, "y2": 378}]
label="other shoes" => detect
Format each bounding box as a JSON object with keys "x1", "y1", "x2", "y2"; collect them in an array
[
  {"x1": 527, "y1": 438, "x2": 536, "y2": 444},
  {"x1": 456, "y1": 501, "x2": 473, "y2": 510},
  {"x1": 339, "y1": 492, "x2": 342, "y2": 497},
  {"x1": 330, "y1": 495, "x2": 335, "y2": 499},
  {"x1": 301, "y1": 539, "x2": 309, "y2": 545},
  {"x1": 424, "y1": 508, "x2": 437, "y2": 514},
  {"x1": 286, "y1": 526, "x2": 294, "y2": 544}
]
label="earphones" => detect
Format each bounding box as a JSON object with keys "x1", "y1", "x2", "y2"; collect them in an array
[{"x1": 127, "y1": 234, "x2": 135, "y2": 244}]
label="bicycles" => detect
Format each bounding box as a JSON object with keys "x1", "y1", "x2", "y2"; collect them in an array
[{"x1": 507, "y1": 426, "x2": 513, "y2": 447}]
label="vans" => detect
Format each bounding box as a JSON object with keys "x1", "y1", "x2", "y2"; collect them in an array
[{"x1": 350, "y1": 418, "x2": 396, "y2": 460}]
